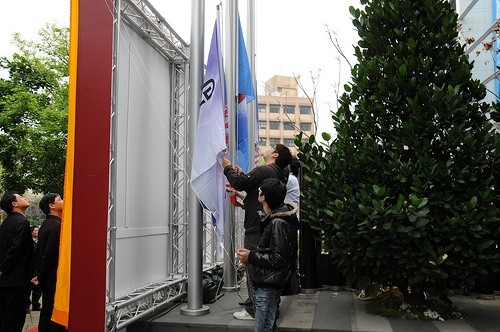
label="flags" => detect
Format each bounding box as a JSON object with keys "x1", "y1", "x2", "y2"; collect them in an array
[
  {"x1": 188, "y1": 4, "x2": 231, "y2": 237},
  {"x1": 231, "y1": 11, "x2": 260, "y2": 207}
]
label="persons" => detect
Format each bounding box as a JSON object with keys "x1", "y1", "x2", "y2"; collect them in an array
[
  {"x1": 237, "y1": 177, "x2": 300, "y2": 332},
  {"x1": 30, "y1": 192, "x2": 67, "y2": 332},
  {"x1": 0, "y1": 190, "x2": 34, "y2": 332},
  {"x1": 222, "y1": 143, "x2": 300, "y2": 319},
  {"x1": 26, "y1": 224, "x2": 42, "y2": 314}
]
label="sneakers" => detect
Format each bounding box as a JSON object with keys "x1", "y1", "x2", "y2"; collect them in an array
[{"x1": 233, "y1": 308, "x2": 256, "y2": 319}]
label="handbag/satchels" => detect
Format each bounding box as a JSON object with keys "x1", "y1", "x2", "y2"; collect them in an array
[{"x1": 280, "y1": 271, "x2": 300, "y2": 296}]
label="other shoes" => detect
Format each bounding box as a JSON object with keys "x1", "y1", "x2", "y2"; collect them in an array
[
  {"x1": 31, "y1": 306, "x2": 42, "y2": 311},
  {"x1": 239, "y1": 302, "x2": 247, "y2": 305}
]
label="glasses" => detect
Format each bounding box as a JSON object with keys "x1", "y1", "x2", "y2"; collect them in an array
[
  {"x1": 271, "y1": 145, "x2": 276, "y2": 151},
  {"x1": 258, "y1": 187, "x2": 263, "y2": 193}
]
